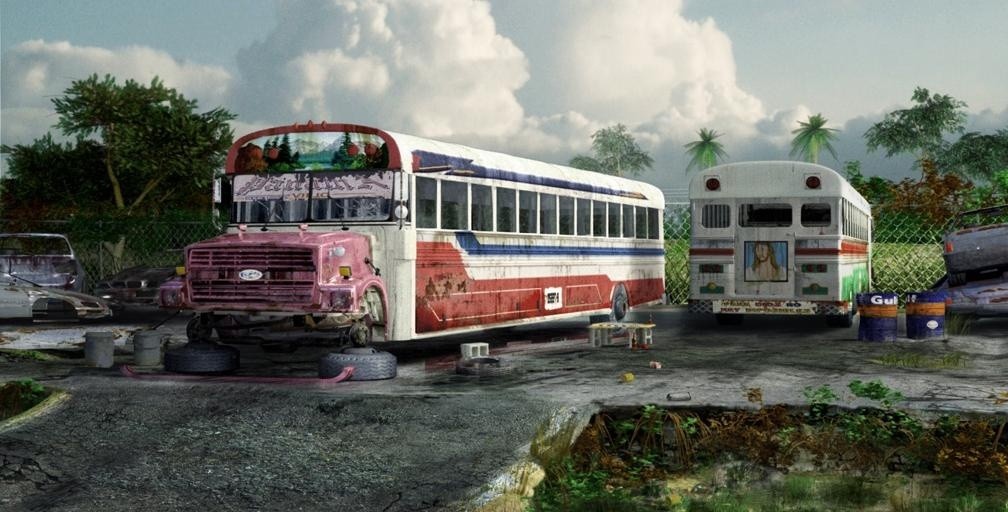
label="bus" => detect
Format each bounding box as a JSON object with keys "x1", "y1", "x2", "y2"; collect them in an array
[
  {"x1": 687, "y1": 160, "x2": 872, "y2": 326},
  {"x1": 158, "y1": 120, "x2": 666, "y2": 349}
]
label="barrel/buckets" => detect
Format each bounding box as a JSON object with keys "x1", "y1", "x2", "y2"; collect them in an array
[
  {"x1": 83, "y1": 332, "x2": 115, "y2": 370},
  {"x1": 904, "y1": 291, "x2": 946, "y2": 338},
  {"x1": 133, "y1": 328, "x2": 163, "y2": 366},
  {"x1": 856, "y1": 292, "x2": 898, "y2": 343}
]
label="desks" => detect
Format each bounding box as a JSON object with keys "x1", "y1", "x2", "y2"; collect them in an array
[{"x1": 589, "y1": 323, "x2": 657, "y2": 349}]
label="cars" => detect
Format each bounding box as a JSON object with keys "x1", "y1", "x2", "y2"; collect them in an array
[
  {"x1": 0, "y1": 232, "x2": 175, "y2": 326},
  {"x1": 942, "y1": 204, "x2": 1008, "y2": 288}
]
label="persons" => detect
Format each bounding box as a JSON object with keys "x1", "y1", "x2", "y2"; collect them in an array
[{"x1": 746, "y1": 243, "x2": 787, "y2": 281}]
